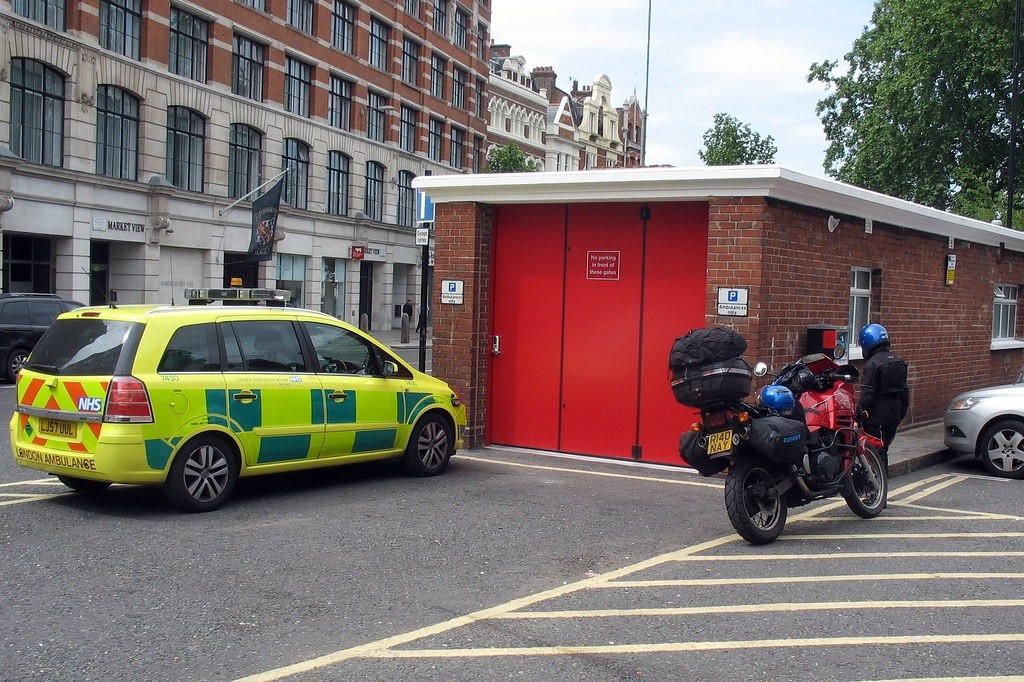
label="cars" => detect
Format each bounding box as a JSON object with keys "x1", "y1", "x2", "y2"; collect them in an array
[{"x1": 942, "y1": 380, "x2": 1024, "y2": 480}]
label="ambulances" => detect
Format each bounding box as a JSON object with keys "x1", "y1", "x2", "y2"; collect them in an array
[{"x1": 6, "y1": 277, "x2": 469, "y2": 513}]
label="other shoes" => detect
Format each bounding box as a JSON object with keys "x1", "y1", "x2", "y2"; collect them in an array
[{"x1": 861, "y1": 498, "x2": 888, "y2": 509}]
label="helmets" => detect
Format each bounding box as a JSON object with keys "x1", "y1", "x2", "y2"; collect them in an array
[
  {"x1": 858, "y1": 324, "x2": 890, "y2": 360},
  {"x1": 760, "y1": 384, "x2": 795, "y2": 414}
]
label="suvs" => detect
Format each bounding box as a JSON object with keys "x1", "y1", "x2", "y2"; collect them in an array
[{"x1": 0, "y1": 293, "x2": 90, "y2": 386}]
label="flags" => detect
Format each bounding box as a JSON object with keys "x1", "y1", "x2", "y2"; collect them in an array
[{"x1": 245, "y1": 176, "x2": 284, "y2": 263}]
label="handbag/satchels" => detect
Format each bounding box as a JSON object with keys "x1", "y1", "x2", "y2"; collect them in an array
[
  {"x1": 679, "y1": 428, "x2": 730, "y2": 477},
  {"x1": 750, "y1": 417, "x2": 806, "y2": 469}
]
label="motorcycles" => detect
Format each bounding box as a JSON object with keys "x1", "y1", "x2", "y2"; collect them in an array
[{"x1": 668, "y1": 343, "x2": 891, "y2": 547}]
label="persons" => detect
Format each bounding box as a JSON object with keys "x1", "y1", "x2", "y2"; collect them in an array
[
  {"x1": 857, "y1": 323, "x2": 910, "y2": 509},
  {"x1": 403, "y1": 300, "x2": 428, "y2": 333}
]
